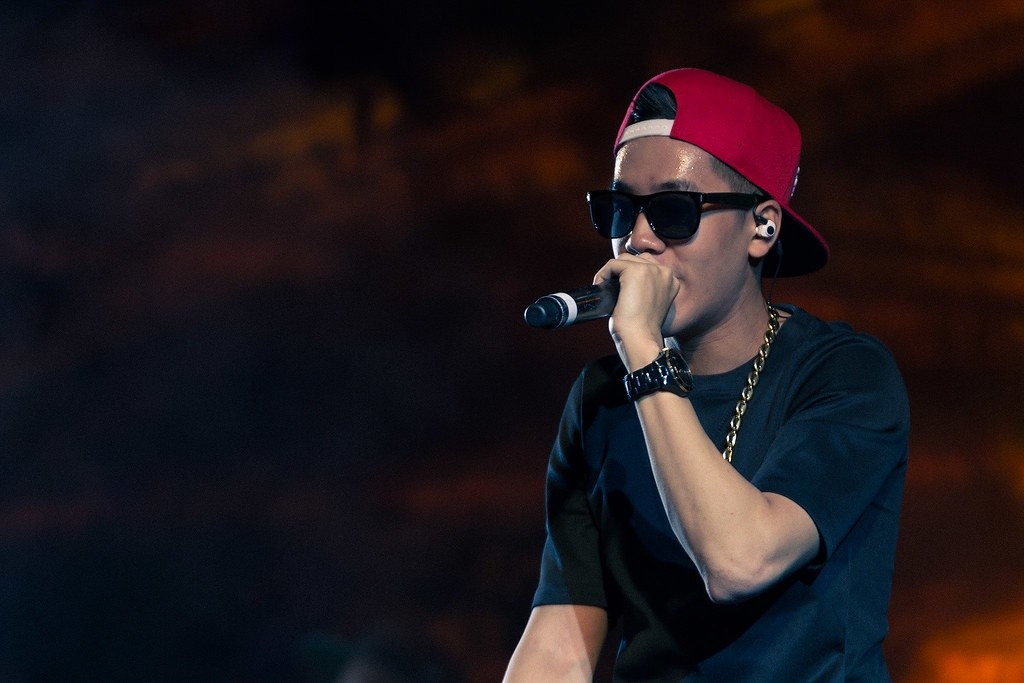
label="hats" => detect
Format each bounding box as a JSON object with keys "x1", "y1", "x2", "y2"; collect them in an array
[{"x1": 614, "y1": 68, "x2": 833, "y2": 278}]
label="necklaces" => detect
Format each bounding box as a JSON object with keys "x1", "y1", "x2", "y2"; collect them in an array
[{"x1": 722, "y1": 300, "x2": 780, "y2": 463}]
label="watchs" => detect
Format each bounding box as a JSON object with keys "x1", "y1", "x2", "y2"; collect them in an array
[{"x1": 623, "y1": 347, "x2": 695, "y2": 401}]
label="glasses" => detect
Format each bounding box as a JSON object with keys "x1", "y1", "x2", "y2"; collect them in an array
[{"x1": 585, "y1": 187, "x2": 764, "y2": 241}]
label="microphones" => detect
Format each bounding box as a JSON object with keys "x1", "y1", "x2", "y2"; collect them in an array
[{"x1": 524, "y1": 276, "x2": 622, "y2": 330}]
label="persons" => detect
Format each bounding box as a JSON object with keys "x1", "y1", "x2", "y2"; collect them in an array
[{"x1": 500, "y1": 67, "x2": 908, "y2": 683}]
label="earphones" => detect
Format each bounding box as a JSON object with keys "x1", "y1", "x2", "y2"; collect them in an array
[{"x1": 756, "y1": 219, "x2": 776, "y2": 238}]
